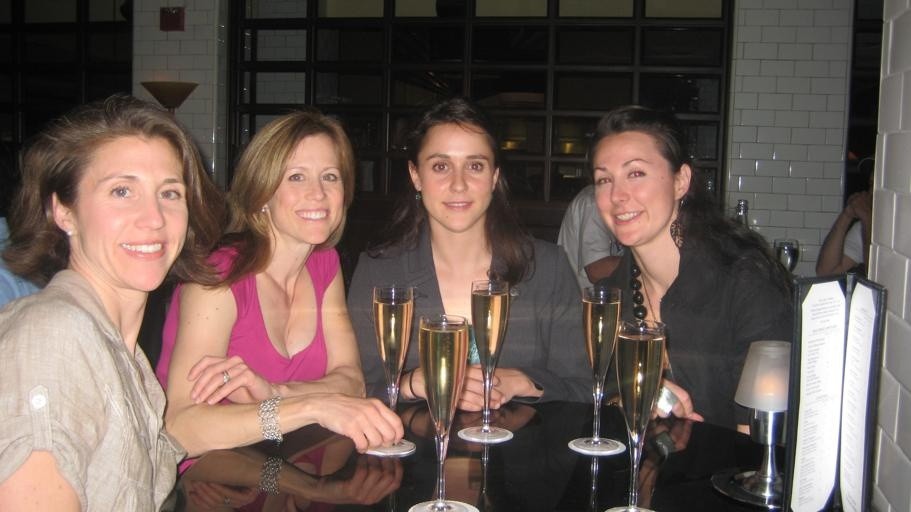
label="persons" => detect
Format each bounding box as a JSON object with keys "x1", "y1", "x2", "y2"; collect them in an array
[
  {"x1": 172, "y1": 436, "x2": 406, "y2": 511},
  {"x1": 844, "y1": 124, "x2": 870, "y2": 206},
  {"x1": 815, "y1": 186, "x2": 873, "y2": 276},
  {"x1": 347, "y1": 96, "x2": 604, "y2": 410},
  {"x1": 561, "y1": 418, "x2": 786, "y2": 511},
  {"x1": 151, "y1": 107, "x2": 406, "y2": 475},
  {"x1": 359, "y1": 403, "x2": 593, "y2": 512},
  {"x1": 0, "y1": 94, "x2": 226, "y2": 512},
  {"x1": 584, "y1": 105, "x2": 801, "y2": 439},
  {"x1": 554, "y1": 179, "x2": 630, "y2": 298},
  {"x1": 0, "y1": 151, "x2": 41, "y2": 313}
]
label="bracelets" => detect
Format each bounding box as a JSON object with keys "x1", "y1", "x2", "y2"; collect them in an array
[
  {"x1": 407, "y1": 366, "x2": 422, "y2": 399},
  {"x1": 407, "y1": 408, "x2": 421, "y2": 433},
  {"x1": 260, "y1": 455, "x2": 286, "y2": 497},
  {"x1": 259, "y1": 393, "x2": 287, "y2": 448}
]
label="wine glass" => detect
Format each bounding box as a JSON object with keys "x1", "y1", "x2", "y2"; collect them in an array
[
  {"x1": 604, "y1": 318, "x2": 667, "y2": 512},
  {"x1": 406, "y1": 311, "x2": 479, "y2": 512},
  {"x1": 362, "y1": 281, "x2": 418, "y2": 459},
  {"x1": 461, "y1": 274, "x2": 517, "y2": 447},
  {"x1": 567, "y1": 283, "x2": 626, "y2": 458},
  {"x1": 470, "y1": 442, "x2": 500, "y2": 510}
]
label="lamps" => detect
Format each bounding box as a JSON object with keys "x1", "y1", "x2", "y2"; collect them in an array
[
  {"x1": 138, "y1": 79, "x2": 202, "y2": 115},
  {"x1": 709, "y1": 337, "x2": 794, "y2": 511}
]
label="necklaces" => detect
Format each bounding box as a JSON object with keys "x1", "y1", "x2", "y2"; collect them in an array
[
  {"x1": 629, "y1": 263, "x2": 662, "y2": 331},
  {"x1": 638, "y1": 460, "x2": 657, "y2": 506}
]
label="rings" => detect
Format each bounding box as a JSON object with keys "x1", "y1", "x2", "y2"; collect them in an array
[
  {"x1": 225, "y1": 497, "x2": 232, "y2": 505},
  {"x1": 222, "y1": 370, "x2": 231, "y2": 382}
]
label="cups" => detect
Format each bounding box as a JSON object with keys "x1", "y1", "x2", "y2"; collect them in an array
[{"x1": 773, "y1": 237, "x2": 802, "y2": 275}]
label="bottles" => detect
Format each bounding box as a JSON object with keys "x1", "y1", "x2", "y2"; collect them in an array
[{"x1": 733, "y1": 198, "x2": 750, "y2": 230}]
